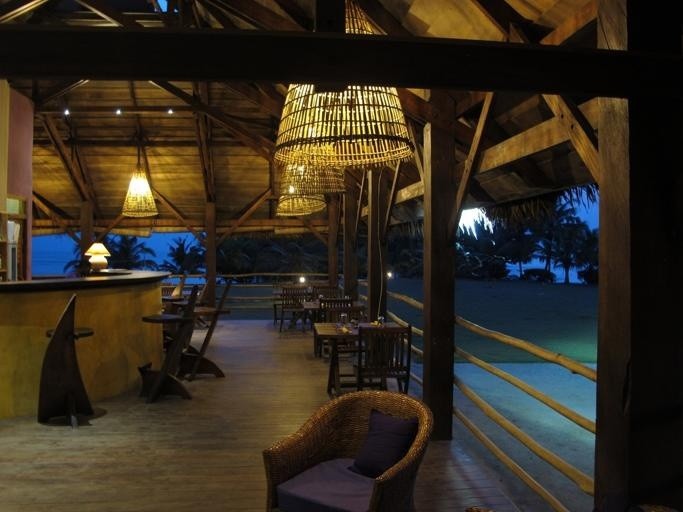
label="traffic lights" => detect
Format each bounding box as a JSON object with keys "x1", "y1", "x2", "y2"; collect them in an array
[{"x1": 348, "y1": 407, "x2": 418, "y2": 479}]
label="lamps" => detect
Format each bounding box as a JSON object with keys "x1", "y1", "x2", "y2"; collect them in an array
[
  {"x1": 262, "y1": 388, "x2": 433, "y2": 512},
  {"x1": 138, "y1": 271, "x2": 232, "y2": 402},
  {"x1": 39, "y1": 293, "x2": 94, "y2": 426},
  {"x1": 272, "y1": 281, "x2": 412, "y2": 398}
]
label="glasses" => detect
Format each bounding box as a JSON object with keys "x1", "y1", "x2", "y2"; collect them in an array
[
  {"x1": 275, "y1": 195, "x2": 326, "y2": 215},
  {"x1": 273, "y1": 0, "x2": 415, "y2": 168},
  {"x1": 279, "y1": 165, "x2": 348, "y2": 194},
  {"x1": 84, "y1": 242, "x2": 110, "y2": 270},
  {"x1": 120, "y1": 141, "x2": 159, "y2": 218}
]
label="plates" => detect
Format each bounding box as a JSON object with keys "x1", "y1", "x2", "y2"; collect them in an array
[{"x1": 1, "y1": 195, "x2": 29, "y2": 282}]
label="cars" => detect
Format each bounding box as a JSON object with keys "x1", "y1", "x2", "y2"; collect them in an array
[{"x1": 338, "y1": 313, "x2": 348, "y2": 323}]
